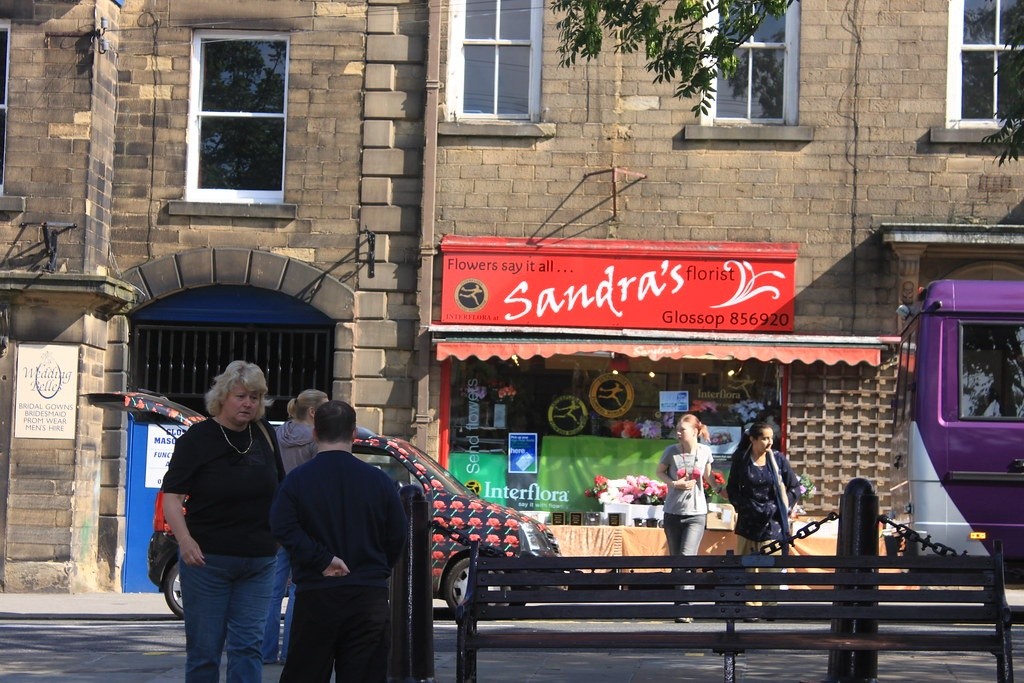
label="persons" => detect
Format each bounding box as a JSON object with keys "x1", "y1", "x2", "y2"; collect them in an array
[
  {"x1": 656, "y1": 415, "x2": 729, "y2": 624},
  {"x1": 161, "y1": 360, "x2": 287, "y2": 683},
  {"x1": 268, "y1": 400, "x2": 407, "y2": 683},
  {"x1": 725, "y1": 421, "x2": 807, "y2": 624},
  {"x1": 260, "y1": 390, "x2": 330, "y2": 666}
]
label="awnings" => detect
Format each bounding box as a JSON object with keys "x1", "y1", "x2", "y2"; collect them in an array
[{"x1": 429, "y1": 332, "x2": 904, "y2": 368}]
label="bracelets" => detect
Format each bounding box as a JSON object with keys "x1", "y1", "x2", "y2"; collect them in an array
[
  {"x1": 673, "y1": 482, "x2": 677, "y2": 489},
  {"x1": 178, "y1": 535, "x2": 189, "y2": 545}
]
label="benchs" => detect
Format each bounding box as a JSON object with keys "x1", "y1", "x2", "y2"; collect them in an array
[{"x1": 455, "y1": 538, "x2": 1015, "y2": 683}]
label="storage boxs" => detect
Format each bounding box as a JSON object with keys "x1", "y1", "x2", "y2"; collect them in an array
[{"x1": 706, "y1": 503, "x2": 735, "y2": 531}]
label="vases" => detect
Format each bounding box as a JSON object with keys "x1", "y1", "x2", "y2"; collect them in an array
[{"x1": 602, "y1": 505, "x2": 662, "y2": 526}]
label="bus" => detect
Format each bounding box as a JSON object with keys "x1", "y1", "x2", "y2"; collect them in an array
[{"x1": 889, "y1": 279, "x2": 1024, "y2": 585}]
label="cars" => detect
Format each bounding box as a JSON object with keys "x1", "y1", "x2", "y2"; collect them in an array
[{"x1": 75, "y1": 393, "x2": 568, "y2": 621}]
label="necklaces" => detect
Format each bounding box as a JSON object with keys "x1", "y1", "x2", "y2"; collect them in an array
[
  {"x1": 217, "y1": 418, "x2": 252, "y2": 454},
  {"x1": 681, "y1": 443, "x2": 698, "y2": 480}
]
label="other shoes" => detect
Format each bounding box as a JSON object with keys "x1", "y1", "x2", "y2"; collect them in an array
[
  {"x1": 264, "y1": 652, "x2": 281, "y2": 663},
  {"x1": 675, "y1": 618, "x2": 693, "y2": 623},
  {"x1": 743, "y1": 618, "x2": 760, "y2": 622}
]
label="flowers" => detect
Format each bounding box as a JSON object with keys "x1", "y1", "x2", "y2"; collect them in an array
[{"x1": 585, "y1": 471, "x2": 816, "y2": 505}]
